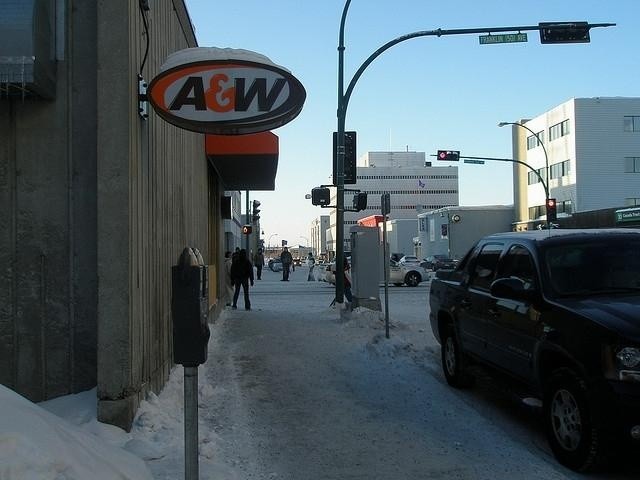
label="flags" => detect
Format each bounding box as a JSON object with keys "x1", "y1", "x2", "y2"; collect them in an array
[{"x1": 419, "y1": 180, "x2": 425, "y2": 189}]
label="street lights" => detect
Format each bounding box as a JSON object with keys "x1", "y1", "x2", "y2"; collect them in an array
[{"x1": 498, "y1": 122, "x2": 550, "y2": 229}]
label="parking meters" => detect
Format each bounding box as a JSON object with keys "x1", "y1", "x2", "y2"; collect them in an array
[{"x1": 171, "y1": 246, "x2": 210, "y2": 480}]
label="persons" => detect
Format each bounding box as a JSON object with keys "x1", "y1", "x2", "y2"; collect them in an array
[
  {"x1": 225, "y1": 251, "x2": 232, "y2": 257},
  {"x1": 230, "y1": 249, "x2": 254, "y2": 310},
  {"x1": 254, "y1": 248, "x2": 265, "y2": 280},
  {"x1": 281, "y1": 247, "x2": 292, "y2": 281},
  {"x1": 232, "y1": 247, "x2": 240, "y2": 256},
  {"x1": 343, "y1": 256, "x2": 352, "y2": 302},
  {"x1": 308, "y1": 252, "x2": 315, "y2": 282}
]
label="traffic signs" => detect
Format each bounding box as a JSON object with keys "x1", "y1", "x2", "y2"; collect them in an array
[{"x1": 479, "y1": 33, "x2": 528, "y2": 44}]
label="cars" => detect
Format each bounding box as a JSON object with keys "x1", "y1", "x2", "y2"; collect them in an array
[
  {"x1": 318, "y1": 250, "x2": 459, "y2": 287},
  {"x1": 268, "y1": 256, "x2": 302, "y2": 272},
  {"x1": 429, "y1": 228, "x2": 640, "y2": 475}
]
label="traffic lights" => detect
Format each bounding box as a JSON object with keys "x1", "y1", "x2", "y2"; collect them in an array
[
  {"x1": 253, "y1": 200, "x2": 260, "y2": 225},
  {"x1": 437, "y1": 150, "x2": 460, "y2": 161},
  {"x1": 546, "y1": 198, "x2": 557, "y2": 221},
  {"x1": 243, "y1": 225, "x2": 252, "y2": 234}
]
